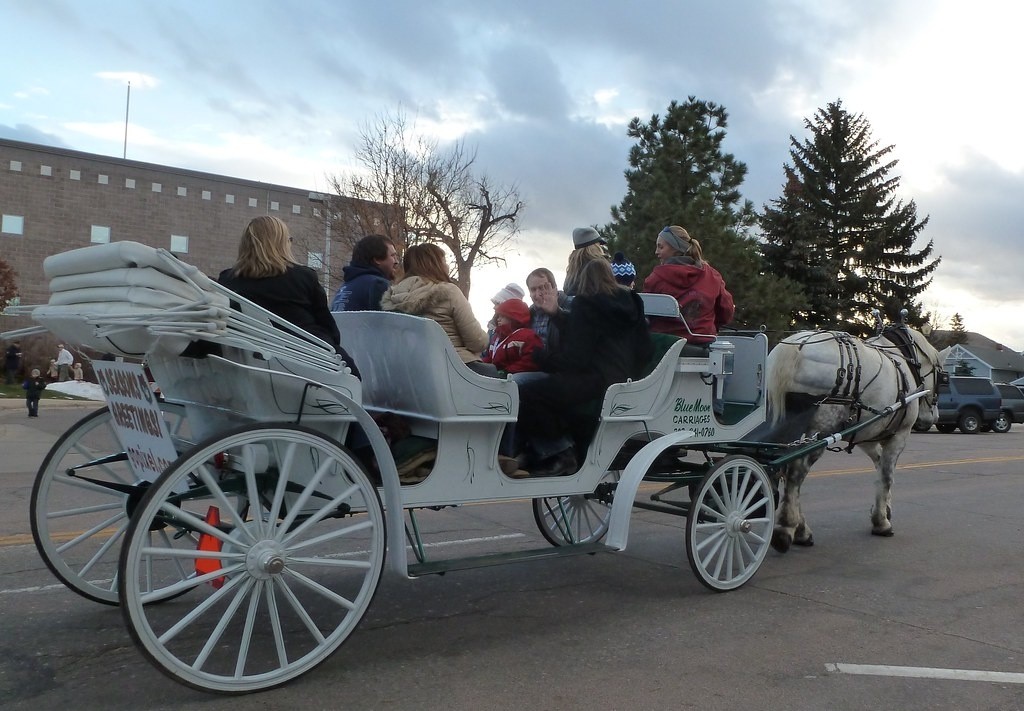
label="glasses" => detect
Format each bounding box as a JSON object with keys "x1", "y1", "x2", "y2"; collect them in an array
[{"x1": 287, "y1": 236, "x2": 294, "y2": 243}]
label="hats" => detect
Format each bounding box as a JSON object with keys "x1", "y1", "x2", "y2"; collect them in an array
[
  {"x1": 32, "y1": 369, "x2": 40, "y2": 376},
  {"x1": 611, "y1": 252, "x2": 636, "y2": 286},
  {"x1": 572, "y1": 226, "x2": 608, "y2": 248},
  {"x1": 57, "y1": 344, "x2": 64, "y2": 347},
  {"x1": 492, "y1": 283, "x2": 525, "y2": 306},
  {"x1": 495, "y1": 299, "x2": 532, "y2": 325}
]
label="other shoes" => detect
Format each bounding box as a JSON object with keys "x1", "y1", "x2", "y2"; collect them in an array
[
  {"x1": 27, "y1": 413, "x2": 38, "y2": 417},
  {"x1": 498, "y1": 454, "x2": 530, "y2": 478}
]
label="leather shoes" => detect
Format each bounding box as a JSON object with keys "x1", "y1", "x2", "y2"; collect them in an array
[
  {"x1": 515, "y1": 447, "x2": 537, "y2": 470},
  {"x1": 531, "y1": 449, "x2": 578, "y2": 477}
]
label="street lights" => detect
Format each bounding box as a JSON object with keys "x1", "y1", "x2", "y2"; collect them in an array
[{"x1": 308, "y1": 192, "x2": 332, "y2": 304}]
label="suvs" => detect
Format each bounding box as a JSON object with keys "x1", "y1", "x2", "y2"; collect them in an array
[
  {"x1": 911, "y1": 376, "x2": 1002, "y2": 434},
  {"x1": 979, "y1": 380, "x2": 1024, "y2": 434}
]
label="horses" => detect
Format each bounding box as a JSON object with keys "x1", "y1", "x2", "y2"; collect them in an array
[{"x1": 766, "y1": 321, "x2": 952, "y2": 556}]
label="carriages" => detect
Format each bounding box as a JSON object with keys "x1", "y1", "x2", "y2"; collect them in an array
[{"x1": 30, "y1": 240, "x2": 952, "y2": 697}]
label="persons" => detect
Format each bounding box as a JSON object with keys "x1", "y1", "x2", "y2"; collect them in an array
[
  {"x1": 499, "y1": 268, "x2": 574, "y2": 456},
  {"x1": 610, "y1": 252, "x2": 656, "y2": 365},
  {"x1": 5, "y1": 339, "x2": 22, "y2": 384},
  {"x1": 217, "y1": 216, "x2": 378, "y2": 471},
  {"x1": 515, "y1": 257, "x2": 636, "y2": 469},
  {"x1": 331, "y1": 235, "x2": 400, "y2": 311},
  {"x1": 380, "y1": 243, "x2": 531, "y2": 477},
  {"x1": 642, "y1": 226, "x2": 734, "y2": 343},
  {"x1": 47, "y1": 343, "x2": 83, "y2": 383},
  {"x1": 563, "y1": 227, "x2": 606, "y2": 296},
  {"x1": 480, "y1": 283, "x2": 544, "y2": 374},
  {"x1": 26, "y1": 368, "x2": 46, "y2": 418}
]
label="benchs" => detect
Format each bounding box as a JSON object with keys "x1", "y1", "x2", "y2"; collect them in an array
[
  {"x1": 330, "y1": 308, "x2": 520, "y2": 467},
  {"x1": 581, "y1": 332, "x2": 687, "y2": 459},
  {"x1": 149, "y1": 295, "x2": 365, "y2": 468},
  {"x1": 640, "y1": 293, "x2": 717, "y2": 375}
]
